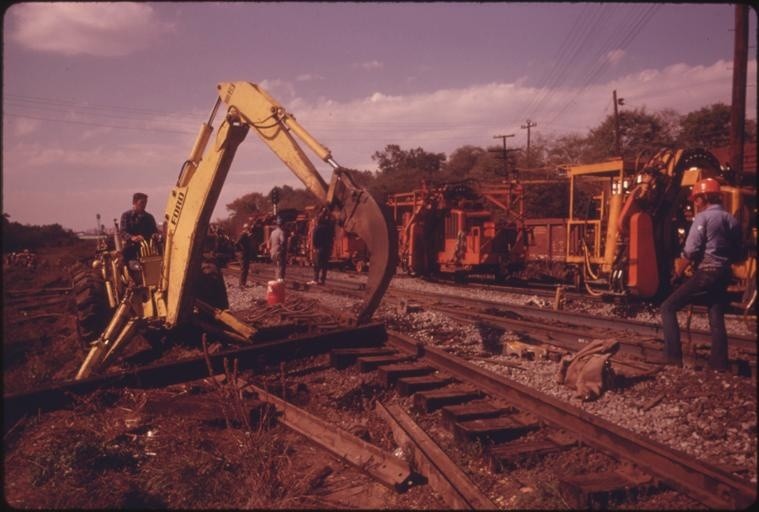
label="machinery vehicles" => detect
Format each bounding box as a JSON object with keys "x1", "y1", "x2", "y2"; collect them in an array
[
  {"x1": 67, "y1": 78, "x2": 400, "y2": 382},
  {"x1": 562, "y1": 147, "x2": 759, "y2": 317}
]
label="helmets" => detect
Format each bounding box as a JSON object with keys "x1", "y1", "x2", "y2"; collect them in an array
[{"x1": 688, "y1": 178, "x2": 721, "y2": 201}]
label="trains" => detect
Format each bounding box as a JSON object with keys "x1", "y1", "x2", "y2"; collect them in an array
[
  {"x1": 240, "y1": 179, "x2": 530, "y2": 283},
  {"x1": 516, "y1": 216, "x2": 595, "y2": 282}
]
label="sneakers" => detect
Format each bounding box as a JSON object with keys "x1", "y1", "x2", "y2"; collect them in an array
[
  {"x1": 306, "y1": 280, "x2": 318, "y2": 285},
  {"x1": 647, "y1": 354, "x2": 682, "y2": 369},
  {"x1": 695, "y1": 363, "x2": 730, "y2": 372},
  {"x1": 319, "y1": 280, "x2": 325, "y2": 285}
]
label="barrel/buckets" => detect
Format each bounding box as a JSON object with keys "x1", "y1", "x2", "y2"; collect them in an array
[{"x1": 266, "y1": 279, "x2": 285, "y2": 307}]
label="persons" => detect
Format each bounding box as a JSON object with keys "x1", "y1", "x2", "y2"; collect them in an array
[
  {"x1": 658, "y1": 177, "x2": 748, "y2": 373},
  {"x1": 117, "y1": 191, "x2": 159, "y2": 261},
  {"x1": 230, "y1": 205, "x2": 336, "y2": 293}
]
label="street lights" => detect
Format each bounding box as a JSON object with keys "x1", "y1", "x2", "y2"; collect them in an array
[{"x1": 521, "y1": 118, "x2": 537, "y2": 168}]
